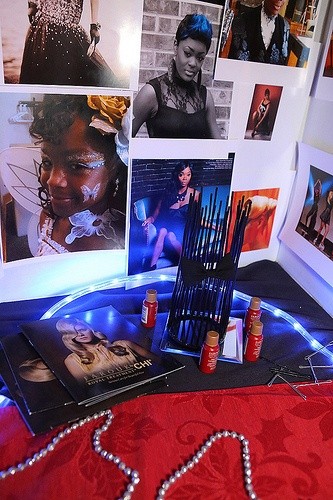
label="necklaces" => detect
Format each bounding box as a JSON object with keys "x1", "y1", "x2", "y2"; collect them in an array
[
  {"x1": 156, "y1": 430, "x2": 257, "y2": 500},
  {"x1": 0, "y1": 410, "x2": 140, "y2": 500}
]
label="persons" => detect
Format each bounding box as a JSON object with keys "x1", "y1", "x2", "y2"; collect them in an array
[
  {"x1": 19, "y1": 0, "x2": 119, "y2": 88},
  {"x1": 18, "y1": 358, "x2": 56, "y2": 382},
  {"x1": 229, "y1": 0, "x2": 291, "y2": 65},
  {"x1": 305, "y1": 179, "x2": 333, "y2": 249},
  {"x1": 56, "y1": 317, "x2": 155, "y2": 382},
  {"x1": 243, "y1": 196, "x2": 277, "y2": 251},
  {"x1": 251, "y1": 89, "x2": 271, "y2": 138},
  {"x1": 142, "y1": 162, "x2": 222, "y2": 271},
  {"x1": 132, "y1": 13, "x2": 221, "y2": 140},
  {"x1": 29, "y1": 93, "x2": 131, "y2": 258}
]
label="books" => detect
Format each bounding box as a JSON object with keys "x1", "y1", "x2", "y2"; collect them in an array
[{"x1": 0, "y1": 305, "x2": 186, "y2": 436}]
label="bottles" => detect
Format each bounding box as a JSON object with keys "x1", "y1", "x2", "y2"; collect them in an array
[
  {"x1": 243, "y1": 321, "x2": 263, "y2": 362},
  {"x1": 243, "y1": 297, "x2": 261, "y2": 334},
  {"x1": 198, "y1": 331, "x2": 220, "y2": 375},
  {"x1": 141, "y1": 289, "x2": 158, "y2": 329}
]
